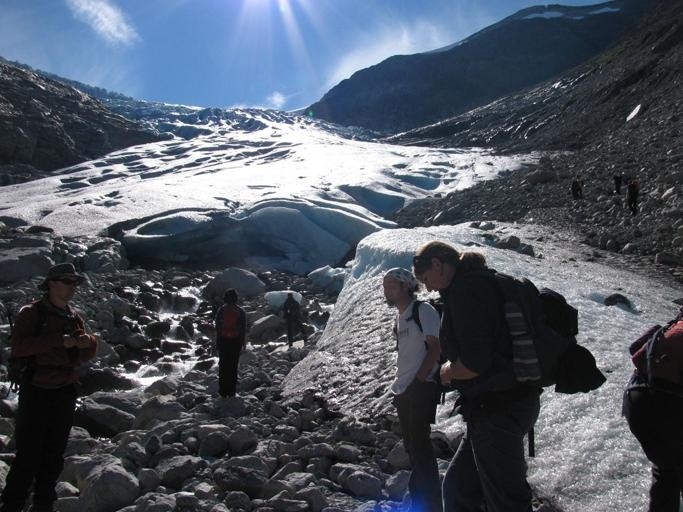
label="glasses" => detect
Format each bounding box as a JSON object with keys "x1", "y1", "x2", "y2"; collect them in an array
[{"x1": 46, "y1": 277, "x2": 83, "y2": 287}]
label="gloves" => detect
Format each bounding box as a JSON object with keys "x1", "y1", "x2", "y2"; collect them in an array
[{"x1": 63, "y1": 330, "x2": 92, "y2": 350}]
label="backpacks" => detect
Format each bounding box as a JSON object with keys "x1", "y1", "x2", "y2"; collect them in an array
[
  {"x1": 493, "y1": 271, "x2": 560, "y2": 387},
  {"x1": 222, "y1": 303, "x2": 243, "y2": 340},
  {"x1": 394, "y1": 298, "x2": 459, "y2": 393}
]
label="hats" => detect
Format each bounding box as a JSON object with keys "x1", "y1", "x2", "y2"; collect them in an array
[{"x1": 37, "y1": 262, "x2": 85, "y2": 291}]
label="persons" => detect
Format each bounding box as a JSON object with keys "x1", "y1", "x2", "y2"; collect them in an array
[
  {"x1": 213, "y1": 286, "x2": 248, "y2": 402},
  {"x1": 612, "y1": 168, "x2": 624, "y2": 193},
  {"x1": 3, "y1": 259, "x2": 100, "y2": 510},
  {"x1": 567, "y1": 175, "x2": 582, "y2": 198},
  {"x1": 625, "y1": 177, "x2": 639, "y2": 213},
  {"x1": 379, "y1": 271, "x2": 445, "y2": 510},
  {"x1": 623, "y1": 307, "x2": 682, "y2": 510},
  {"x1": 411, "y1": 244, "x2": 545, "y2": 510},
  {"x1": 283, "y1": 293, "x2": 305, "y2": 347}
]
